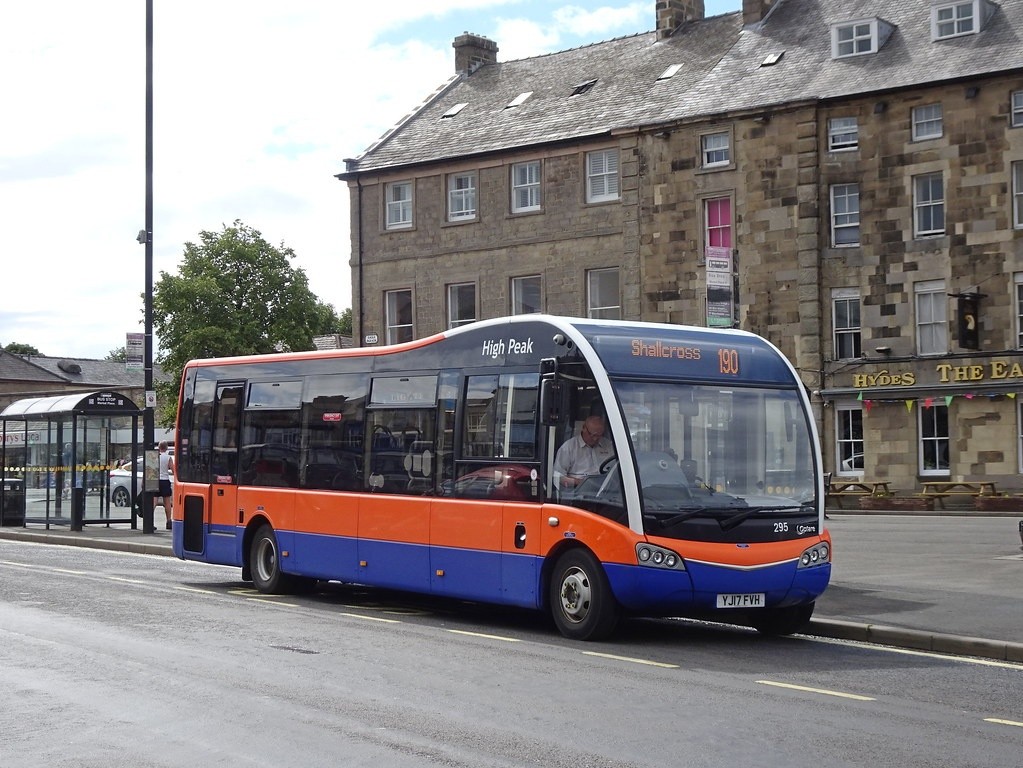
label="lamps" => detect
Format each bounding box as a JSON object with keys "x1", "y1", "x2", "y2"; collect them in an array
[
  {"x1": 654, "y1": 132, "x2": 671, "y2": 140},
  {"x1": 875, "y1": 346, "x2": 892, "y2": 354},
  {"x1": 873, "y1": 101, "x2": 888, "y2": 114},
  {"x1": 965, "y1": 87, "x2": 980, "y2": 99},
  {"x1": 753, "y1": 117, "x2": 770, "y2": 125}
]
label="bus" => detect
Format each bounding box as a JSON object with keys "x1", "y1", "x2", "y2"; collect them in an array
[{"x1": 171, "y1": 313, "x2": 832, "y2": 643}]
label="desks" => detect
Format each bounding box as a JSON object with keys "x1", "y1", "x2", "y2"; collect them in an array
[
  {"x1": 831, "y1": 481, "x2": 892, "y2": 510},
  {"x1": 921, "y1": 481, "x2": 999, "y2": 510}
]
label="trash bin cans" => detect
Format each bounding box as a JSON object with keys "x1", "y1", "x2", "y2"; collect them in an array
[{"x1": 0, "y1": 478, "x2": 23, "y2": 527}]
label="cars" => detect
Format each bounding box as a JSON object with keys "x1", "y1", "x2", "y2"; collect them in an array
[
  {"x1": 102, "y1": 457, "x2": 175, "y2": 507},
  {"x1": 38, "y1": 444, "x2": 188, "y2": 489},
  {"x1": 841, "y1": 452, "x2": 865, "y2": 472}
]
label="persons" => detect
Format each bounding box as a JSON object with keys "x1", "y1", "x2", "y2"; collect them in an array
[
  {"x1": 153, "y1": 441, "x2": 174, "y2": 529},
  {"x1": 554, "y1": 416, "x2": 616, "y2": 492}
]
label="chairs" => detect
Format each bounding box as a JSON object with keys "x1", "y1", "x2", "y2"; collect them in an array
[{"x1": 255, "y1": 418, "x2": 425, "y2": 492}]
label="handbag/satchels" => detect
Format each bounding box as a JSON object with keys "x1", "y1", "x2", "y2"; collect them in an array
[{"x1": 136, "y1": 491, "x2": 144, "y2": 518}]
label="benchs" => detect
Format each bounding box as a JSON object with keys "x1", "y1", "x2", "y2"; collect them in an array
[
  {"x1": 825, "y1": 490, "x2": 900, "y2": 511},
  {"x1": 914, "y1": 490, "x2": 1008, "y2": 510}
]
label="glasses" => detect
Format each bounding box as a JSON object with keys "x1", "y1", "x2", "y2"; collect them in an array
[{"x1": 584, "y1": 425, "x2": 604, "y2": 440}]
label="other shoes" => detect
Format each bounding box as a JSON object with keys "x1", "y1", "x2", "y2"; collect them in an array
[
  {"x1": 166, "y1": 521, "x2": 172, "y2": 529},
  {"x1": 153, "y1": 525, "x2": 157, "y2": 531}
]
